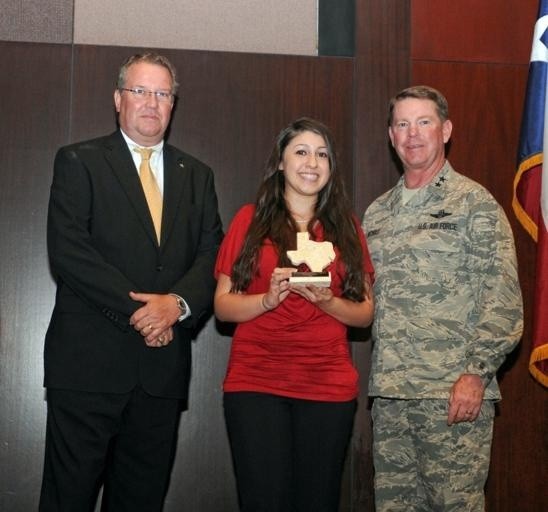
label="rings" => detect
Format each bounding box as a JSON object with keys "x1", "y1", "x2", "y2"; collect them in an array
[
  {"x1": 157, "y1": 336, "x2": 165, "y2": 342},
  {"x1": 147, "y1": 322, "x2": 155, "y2": 331}
]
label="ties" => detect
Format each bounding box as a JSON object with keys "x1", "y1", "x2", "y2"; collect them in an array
[{"x1": 134, "y1": 147, "x2": 164, "y2": 247}]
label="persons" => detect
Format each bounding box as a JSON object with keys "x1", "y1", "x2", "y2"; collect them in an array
[
  {"x1": 361, "y1": 83, "x2": 525, "y2": 511},
  {"x1": 44, "y1": 51, "x2": 223, "y2": 503},
  {"x1": 214, "y1": 115, "x2": 375, "y2": 505}
]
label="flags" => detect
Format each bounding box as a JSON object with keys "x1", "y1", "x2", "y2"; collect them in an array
[{"x1": 509, "y1": 1, "x2": 548, "y2": 386}]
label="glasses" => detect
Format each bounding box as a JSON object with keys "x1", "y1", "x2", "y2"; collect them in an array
[{"x1": 117, "y1": 87, "x2": 175, "y2": 102}]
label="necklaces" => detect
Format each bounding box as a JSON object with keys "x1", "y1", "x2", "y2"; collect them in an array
[{"x1": 291, "y1": 215, "x2": 311, "y2": 225}]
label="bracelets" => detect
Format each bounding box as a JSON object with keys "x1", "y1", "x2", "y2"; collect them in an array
[{"x1": 168, "y1": 290, "x2": 187, "y2": 316}]
